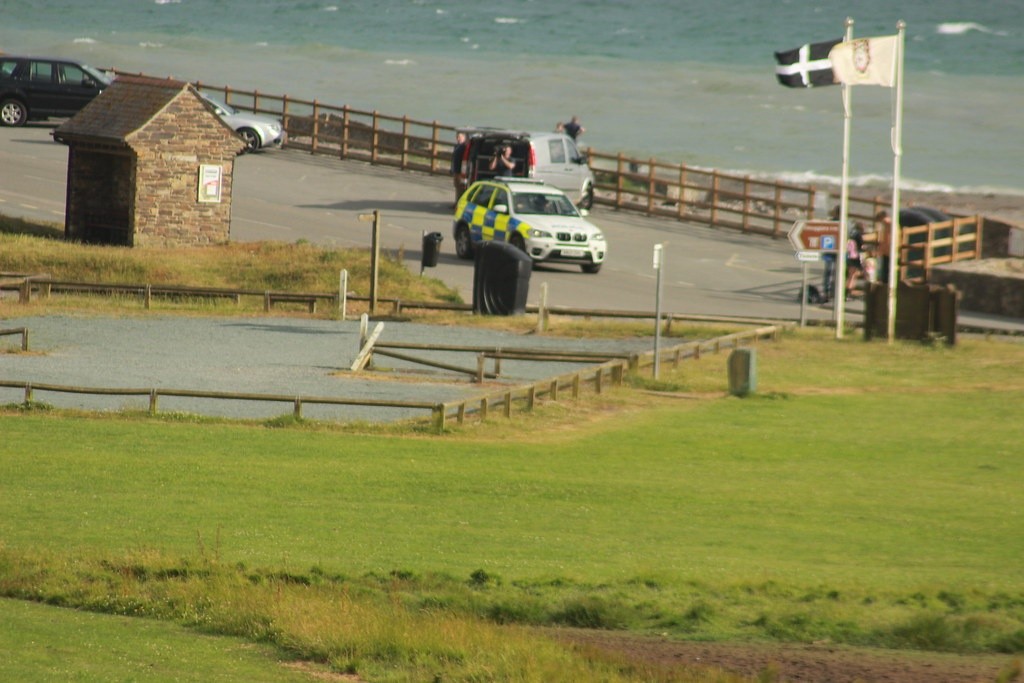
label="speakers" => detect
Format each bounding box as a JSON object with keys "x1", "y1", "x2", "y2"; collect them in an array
[{"x1": 471, "y1": 240, "x2": 532, "y2": 317}]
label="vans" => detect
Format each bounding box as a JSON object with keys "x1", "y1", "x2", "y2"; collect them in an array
[{"x1": 458, "y1": 130, "x2": 596, "y2": 212}]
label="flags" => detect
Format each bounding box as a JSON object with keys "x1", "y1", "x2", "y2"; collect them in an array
[
  {"x1": 828, "y1": 31, "x2": 900, "y2": 88},
  {"x1": 775, "y1": 41, "x2": 838, "y2": 90}
]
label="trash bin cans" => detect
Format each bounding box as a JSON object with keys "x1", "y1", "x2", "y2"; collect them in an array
[
  {"x1": 473, "y1": 239, "x2": 532, "y2": 317},
  {"x1": 424, "y1": 231, "x2": 443, "y2": 266},
  {"x1": 901, "y1": 206, "x2": 950, "y2": 276},
  {"x1": 865, "y1": 282, "x2": 955, "y2": 349}
]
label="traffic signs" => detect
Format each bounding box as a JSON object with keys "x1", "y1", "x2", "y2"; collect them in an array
[{"x1": 787, "y1": 218, "x2": 840, "y2": 254}]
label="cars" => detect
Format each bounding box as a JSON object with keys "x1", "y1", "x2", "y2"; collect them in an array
[
  {"x1": 200, "y1": 93, "x2": 283, "y2": 154},
  {"x1": 451, "y1": 176, "x2": 606, "y2": 274}
]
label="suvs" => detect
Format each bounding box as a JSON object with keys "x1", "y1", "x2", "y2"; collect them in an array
[{"x1": 0, "y1": 53, "x2": 113, "y2": 128}]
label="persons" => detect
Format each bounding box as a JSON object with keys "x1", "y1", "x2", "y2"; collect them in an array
[
  {"x1": 846, "y1": 226, "x2": 864, "y2": 302},
  {"x1": 447, "y1": 132, "x2": 471, "y2": 209},
  {"x1": 823, "y1": 201, "x2": 851, "y2": 302},
  {"x1": 563, "y1": 114, "x2": 584, "y2": 140},
  {"x1": 489, "y1": 144, "x2": 516, "y2": 176},
  {"x1": 534, "y1": 195, "x2": 549, "y2": 214},
  {"x1": 554, "y1": 122, "x2": 564, "y2": 133},
  {"x1": 876, "y1": 210, "x2": 902, "y2": 284}
]
